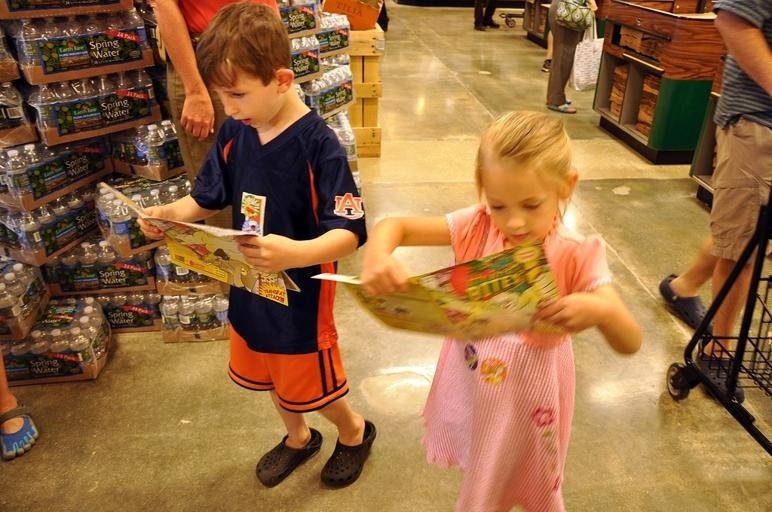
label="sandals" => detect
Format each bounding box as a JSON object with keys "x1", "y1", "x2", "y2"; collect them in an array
[{"x1": 545, "y1": 100, "x2": 577, "y2": 113}]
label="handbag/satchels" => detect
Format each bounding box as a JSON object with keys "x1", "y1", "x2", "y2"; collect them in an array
[
  {"x1": 568, "y1": 38, "x2": 606, "y2": 92},
  {"x1": 555, "y1": 0, "x2": 593, "y2": 31}
]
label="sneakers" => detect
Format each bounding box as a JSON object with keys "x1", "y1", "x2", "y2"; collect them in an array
[
  {"x1": 541, "y1": 58, "x2": 552, "y2": 72},
  {"x1": 1, "y1": 394, "x2": 40, "y2": 460}
]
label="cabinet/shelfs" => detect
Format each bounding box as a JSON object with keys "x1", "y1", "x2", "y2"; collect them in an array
[
  {"x1": 687, "y1": 45, "x2": 728, "y2": 209},
  {"x1": 590, "y1": 0, "x2": 725, "y2": 166}
]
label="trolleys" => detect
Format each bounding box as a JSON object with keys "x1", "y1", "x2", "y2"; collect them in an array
[{"x1": 663, "y1": 167, "x2": 772, "y2": 459}]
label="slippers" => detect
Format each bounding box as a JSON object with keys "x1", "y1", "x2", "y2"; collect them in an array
[
  {"x1": 697, "y1": 337, "x2": 745, "y2": 405},
  {"x1": 321, "y1": 421, "x2": 376, "y2": 488},
  {"x1": 660, "y1": 273, "x2": 714, "y2": 331},
  {"x1": 256, "y1": 428, "x2": 322, "y2": 488}
]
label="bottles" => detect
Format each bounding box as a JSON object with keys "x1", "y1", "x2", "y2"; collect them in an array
[
  {"x1": 0, "y1": 8, "x2": 154, "y2": 69},
  {"x1": 0, "y1": 69, "x2": 193, "y2": 181},
  {"x1": 0, "y1": 182, "x2": 231, "y2": 386},
  {"x1": 316, "y1": 12, "x2": 350, "y2": 57},
  {"x1": 297, "y1": 63, "x2": 355, "y2": 118},
  {"x1": 326, "y1": 111, "x2": 359, "y2": 192},
  {"x1": 277, "y1": 0, "x2": 322, "y2": 38},
  {"x1": 290, "y1": 36, "x2": 323, "y2": 83}
]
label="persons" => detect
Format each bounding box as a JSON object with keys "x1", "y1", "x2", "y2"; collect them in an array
[
  {"x1": 1, "y1": 342, "x2": 39, "y2": 461},
  {"x1": 543, "y1": 24, "x2": 554, "y2": 73},
  {"x1": 147, "y1": 0, "x2": 282, "y2": 231},
  {"x1": 137, "y1": 4, "x2": 376, "y2": 488},
  {"x1": 474, "y1": 1, "x2": 500, "y2": 31},
  {"x1": 657, "y1": 0, "x2": 772, "y2": 404},
  {"x1": 548, "y1": 0, "x2": 599, "y2": 114},
  {"x1": 360, "y1": 109, "x2": 642, "y2": 511}
]
